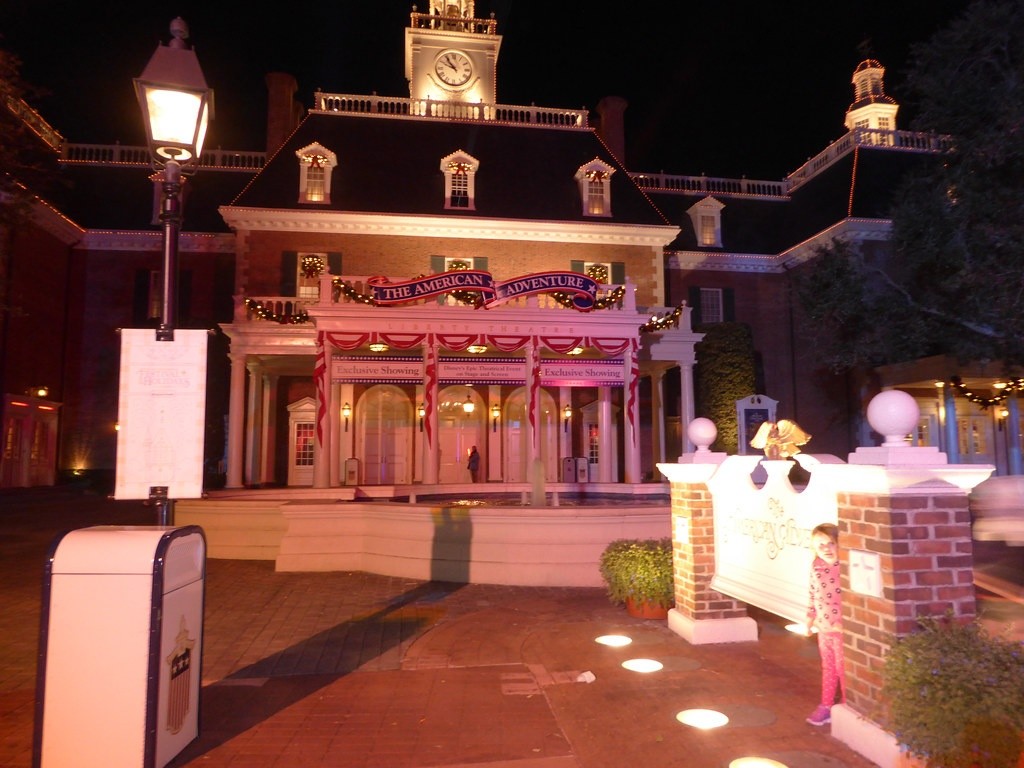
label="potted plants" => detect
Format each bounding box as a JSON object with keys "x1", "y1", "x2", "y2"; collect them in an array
[
  {"x1": 596, "y1": 535, "x2": 677, "y2": 623},
  {"x1": 849, "y1": 608, "x2": 1024, "y2": 768}
]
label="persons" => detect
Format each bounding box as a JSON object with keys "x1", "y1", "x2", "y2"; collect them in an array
[
  {"x1": 467, "y1": 445, "x2": 480, "y2": 483},
  {"x1": 804, "y1": 521, "x2": 846, "y2": 725}
]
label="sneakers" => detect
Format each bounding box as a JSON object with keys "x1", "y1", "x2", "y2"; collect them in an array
[{"x1": 806, "y1": 704, "x2": 832, "y2": 726}]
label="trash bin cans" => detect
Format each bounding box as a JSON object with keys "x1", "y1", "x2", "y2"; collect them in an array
[{"x1": 32, "y1": 522, "x2": 207, "y2": 768}]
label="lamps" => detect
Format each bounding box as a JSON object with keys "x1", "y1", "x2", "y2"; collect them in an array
[
  {"x1": 566, "y1": 346, "x2": 584, "y2": 357},
  {"x1": 562, "y1": 404, "x2": 573, "y2": 433},
  {"x1": 369, "y1": 343, "x2": 390, "y2": 355},
  {"x1": 417, "y1": 402, "x2": 425, "y2": 433},
  {"x1": 462, "y1": 383, "x2": 475, "y2": 420},
  {"x1": 465, "y1": 344, "x2": 488, "y2": 355},
  {"x1": 489, "y1": 403, "x2": 501, "y2": 434},
  {"x1": 341, "y1": 401, "x2": 352, "y2": 433}
]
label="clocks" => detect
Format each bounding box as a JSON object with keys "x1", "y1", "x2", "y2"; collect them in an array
[{"x1": 433, "y1": 51, "x2": 472, "y2": 87}]
